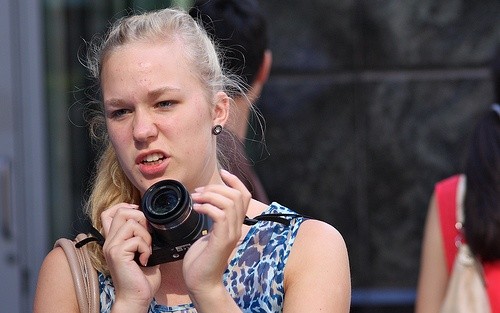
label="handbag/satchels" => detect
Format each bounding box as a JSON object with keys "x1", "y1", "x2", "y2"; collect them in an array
[{"x1": 437, "y1": 243, "x2": 492, "y2": 313}]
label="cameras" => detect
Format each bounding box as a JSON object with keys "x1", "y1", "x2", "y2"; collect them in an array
[{"x1": 134, "y1": 179, "x2": 209, "y2": 268}]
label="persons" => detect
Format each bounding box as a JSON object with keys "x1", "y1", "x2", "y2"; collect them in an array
[
  {"x1": 413, "y1": 103, "x2": 500, "y2": 312},
  {"x1": 33, "y1": 6, "x2": 352, "y2": 313},
  {"x1": 189, "y1": 2, "x2": 274, "y2": 206}
]
